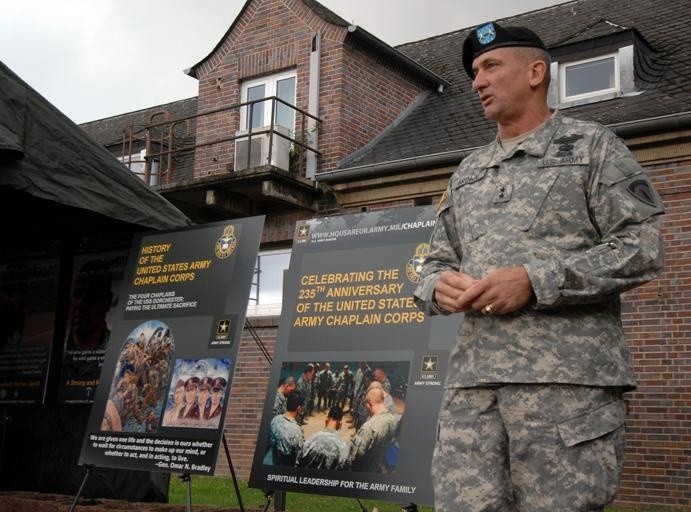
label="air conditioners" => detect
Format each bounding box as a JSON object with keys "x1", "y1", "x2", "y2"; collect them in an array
[{"x1": 233, "y1": 124, "x2": 291, "y2": 172}]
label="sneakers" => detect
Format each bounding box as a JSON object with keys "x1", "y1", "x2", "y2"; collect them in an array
[{"x1": 302, "y1": 406, "x2": 356, "y2": 438}]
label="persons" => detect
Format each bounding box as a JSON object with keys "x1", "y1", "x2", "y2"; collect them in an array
[
  {"x1": 191, "y1": 377, "x2": 214, "y2": 420},
  {"x1": 110, "y1": 326, "x2": 172, "y2": 432},
  {"x1": 206, "y1": 375, "x2": 227, "y2": 418},
  {"x1": 165, "y1": 379, "x2": 184, "y2": 426},
  {"x1": 268, "y1": 362, "x2": 402, "y2": 476},
  {"x1": 178, "y1": 376, "x2": 201, "y2": 420},
  {"x1": 412, "y1": 21, "x2": 666, "y2": 512}
]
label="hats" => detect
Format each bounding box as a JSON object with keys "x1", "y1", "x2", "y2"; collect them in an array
[
  {"x1": 462, "y1": 21, "x2": 550, "y2": 81},
  {"x1": 184, "y1": 375, "x2": 226, "y2": 391}
]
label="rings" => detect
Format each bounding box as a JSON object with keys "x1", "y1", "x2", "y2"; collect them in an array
[{"x1": 481, "y1": 304, "x2": 497, "y2": 319}]
